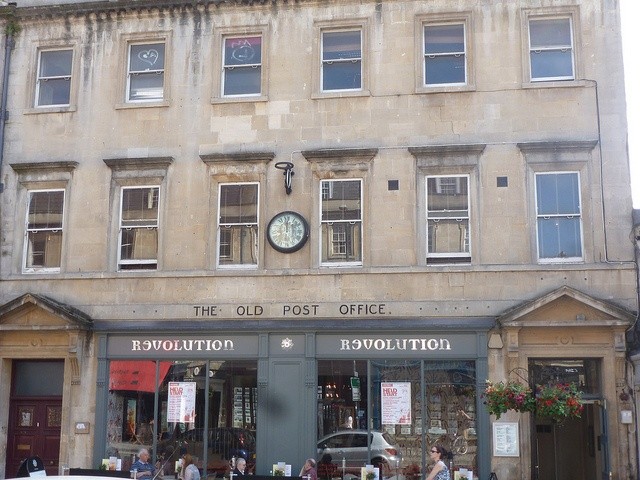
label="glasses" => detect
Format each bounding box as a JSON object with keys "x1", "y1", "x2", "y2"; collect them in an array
[{"x1": 430, "y1": 450, "x2": 440, "y2": 453}]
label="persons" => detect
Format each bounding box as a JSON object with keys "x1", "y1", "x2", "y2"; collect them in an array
[
  {"x1": 298, "y1": 458, "x2": 317, "y2": 480},
  {"x1": 130, "y1": 448, "x2": 155, "y2": 480},
  {"x1": 178, "y1": 454, "x2": 200, "y2": 480},
  {"x1": 456, "y1": 407, "x2": 470, "y2": 453},
  {"x1": 425, "y1": 445, "x2": 450, "y2": 480},
  {"x1": 232, "y1": 458, "x2": 247, "y2": 480}
]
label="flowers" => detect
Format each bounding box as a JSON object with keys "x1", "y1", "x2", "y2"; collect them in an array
[
  {"x1": 479, "y1": 382, "x2": 536, "y2": 421},
  {"x1": 531, "y1": 380, "x2": 587, "y2": 430}
]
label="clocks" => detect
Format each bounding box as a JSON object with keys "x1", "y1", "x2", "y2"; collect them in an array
[{"x1": 266, "y1": 211, "x2": 309, "y2": 253}]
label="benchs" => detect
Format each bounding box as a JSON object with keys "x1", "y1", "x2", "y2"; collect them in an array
[
  {"x1": 59, "y1": 465, "x2": 138, "y2": 480},
  {"x1": 228, "y1": 469, "x2": 313, "y2": 479}
]
